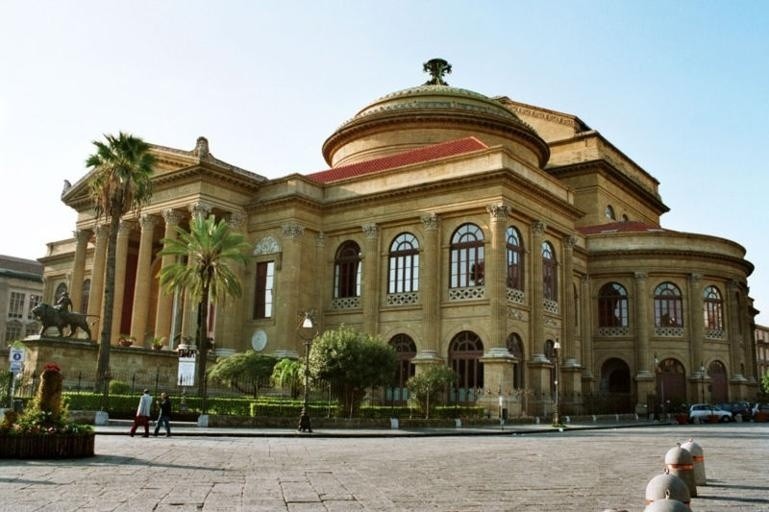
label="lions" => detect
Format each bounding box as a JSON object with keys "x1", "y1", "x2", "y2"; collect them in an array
[{"x1": 32, "y1": 302, "x2": 100, "y2": 340}]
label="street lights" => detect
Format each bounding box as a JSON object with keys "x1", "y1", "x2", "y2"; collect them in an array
[
  {"x1": 297, "y1": 309, "x2": 319, "y2": 432},
  {"x1": 700, "y1": 361, "x2": 704, "y2": 403},
  {"x1": 654, "y1": 352, "x2": 663, "y2": 402},
  {"x1": 551, "y1": 340, "x2": 565, "y2": 427}
]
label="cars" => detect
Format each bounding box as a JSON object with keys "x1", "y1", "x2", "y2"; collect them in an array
[{"x1": 689, "y1": 400, "x2": 769, "y2": 424}]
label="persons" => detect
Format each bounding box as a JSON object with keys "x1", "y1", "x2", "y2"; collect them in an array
[
  {"x1": 152, "y1": 392, "x2": 171, "y2": 437},
  {"x1": 128, "y1": 389, "x2": 153, "y2": 437},
  {"x1": 53, "y1": 292, "x2": 73, "y2": 328}
]
